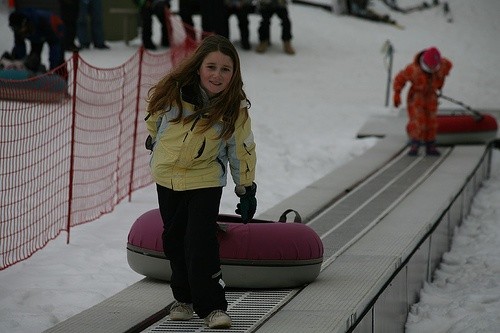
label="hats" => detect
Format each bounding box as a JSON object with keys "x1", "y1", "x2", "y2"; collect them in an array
[
  {"x1": 426, "y1": 49, "x2": 441, "y2": 73},
  {"x1": 8, "y1": 10, "x2": 30, "y2": 28}
]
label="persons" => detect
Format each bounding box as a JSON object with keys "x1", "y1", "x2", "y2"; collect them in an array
[
  {"x1": 393, "y1": 48, "x2": 453, "y2": 157},
  {"x1": 144, "y1": 34, "x2": 257, "y2": 329},
  {"x1": 8, "y1": 0, "x2": 110, "y2": 96},
  {"x1": 139, "y1": 0, "x2": 296, "y2": 54}
]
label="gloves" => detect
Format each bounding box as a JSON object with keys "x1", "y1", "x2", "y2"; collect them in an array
[
  {"x1": 393, "y1": 93, "x2": 402, "y2": 108},
  {"x1": 236, "y1": 182, "x2": 261, "y2": 222},
  {"x1": 144, "y1": 133, "x2": 157, "y2": 151}
]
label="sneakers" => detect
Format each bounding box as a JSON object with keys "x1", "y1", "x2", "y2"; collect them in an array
[
  {"x1": 168, "y1": 299, "x2": 193, "y2": 319},
  {"x1": 204, "y1": 309, "x2": 236, "y2": 329}
]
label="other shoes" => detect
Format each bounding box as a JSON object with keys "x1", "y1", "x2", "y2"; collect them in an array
[
  {"x1": 255, "y1": 40, "x2": 271, "y2": 53},
  {"x1": 425, "y1": 139, "x2": 441, "y2": 156},
  {"x1": 240, "y1": 39, "x2": 252, "y2": 51},
  {"x1": 92, "y1": 41, "x2": 111, "y2": 50},
  {"x1": 161, "y1": 34, "x2": 177, "y2": 48},
  {"x1": 142, "y1": 39, "x2": 158, "y2": 50},
  {"x1": 283, "y1": 40, "x2": 296, "y2": 54},
  {"x1": 63, "y1": 42, "x2": 77, "y2": 52},
  {"x1": 408, "y1": 141, "x2": 422, "y2": 155},
  {"x1": 78, "y1": 41, "x2": 92, "y2": 50}
]
